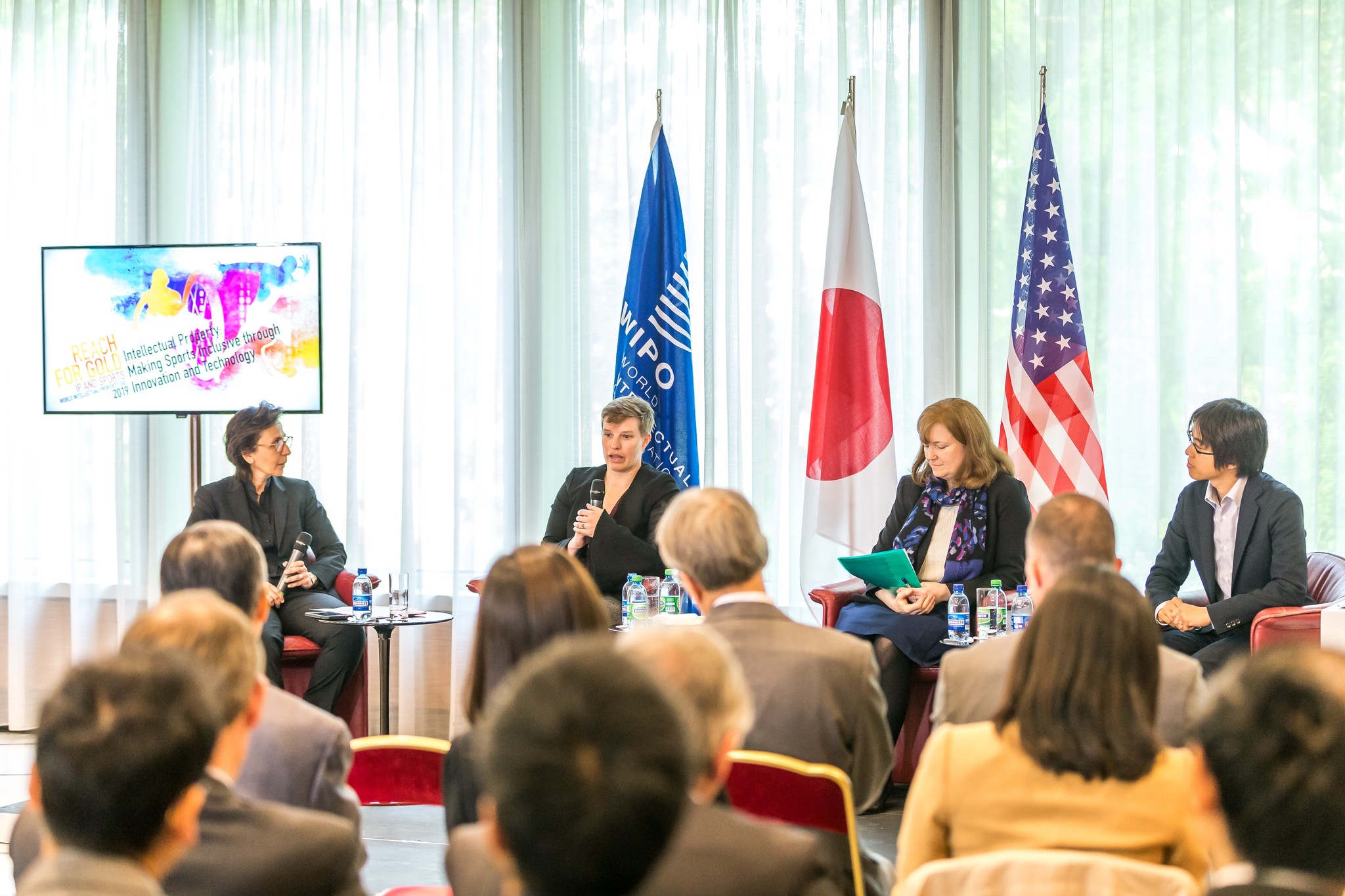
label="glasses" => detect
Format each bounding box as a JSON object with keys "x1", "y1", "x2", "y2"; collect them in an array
[
  {"x1": 1187, "y1": 429, "x2": 1217, "y2": 455},
  {"x1": 253, "y1": 436, "x2": 293, "y2": 453}
]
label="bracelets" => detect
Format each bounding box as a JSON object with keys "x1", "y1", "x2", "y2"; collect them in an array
[{"x1": 307, "y1": 572, "x2": 318, "y2": 588}]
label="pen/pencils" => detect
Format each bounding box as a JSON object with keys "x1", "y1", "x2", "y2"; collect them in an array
[{"x1": 903, "y1": 577, "x2": 911, "y2": 588}]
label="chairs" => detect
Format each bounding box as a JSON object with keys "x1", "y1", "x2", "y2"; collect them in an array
[
  {"x1": 810, "y1": 559, "x2": 1122, "y2": 783},
  {"x1": 723, "y1": 750, "x2": 867, "y2": 896},
  {"x1": 1177, "y1": 552, "x2": 1345, "y2": 662},
  {"x1": 892, "y1": 851, "x2": 1200, "y2": 896},
  {"x1": 281, "y1": 546, "x2": 382, "y2": 739},
  {"x1": 338, "y1": 735, "x2": 451, "y2": 808}
]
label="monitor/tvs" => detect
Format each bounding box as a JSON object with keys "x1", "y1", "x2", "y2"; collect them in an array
[{"x1": 40, "y1": 242, "x2": 323, "y2": 414}]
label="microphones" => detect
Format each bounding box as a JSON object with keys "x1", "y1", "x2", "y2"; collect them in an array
[
  {"x1": 276, "y1": 532, "x2": 313, "y2": 597},
  {"x1": 589, "y1": 480, "x2": 605, "y2": 509}
]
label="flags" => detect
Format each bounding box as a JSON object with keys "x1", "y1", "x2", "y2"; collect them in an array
[
  {"x1": 1000, "y1": 102, "x2": 1110, "y2": 514},
  {"x1": 803, "y1": 96, "x2": 895, "y2": 627}
]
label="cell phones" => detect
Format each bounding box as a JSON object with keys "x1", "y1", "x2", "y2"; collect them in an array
[{"x1": 305, "y1": 611, "x2": 349, "y2": 620}]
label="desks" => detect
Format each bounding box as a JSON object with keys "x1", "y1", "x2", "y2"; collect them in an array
[{"x1": 316, "y1": 605, "x2": 454, "y2": 735}]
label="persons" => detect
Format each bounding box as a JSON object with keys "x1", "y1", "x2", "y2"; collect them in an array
[
  {"x1": 1183, "y1": 672, "x2": 1345, "y2": 896},
  {"x1": 613, "y1": 114, "x2": 700, "y2": 614},
  {"x1": 892, "y1": 565, "x2": 1236, "y2": 880},
  {"x1": 442, "y1": 487, "x2": 895, "y2": 896},
  {"x1": 9, "y1": 519, "x2": 365, "y2": 896},
  {"x1": 541, "y1": 396, "x2": 683, "y2": 629},
  {"x1": 1144, "y1": 402, "x2": 1317, "y2": 679},
  {"x1": 929, "y1": 499, "x2": 1209, "y2": 750},
  {"x1": 182, "y1": 402, "x2": 368, "y2": 715},
  {"x1": 834, "y1": 398, "x2": 1033, "y2": 815}
]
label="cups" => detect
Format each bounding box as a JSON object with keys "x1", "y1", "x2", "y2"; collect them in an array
[
  {"x1": 642, "y1": 576, "x2": 660, "y2": 619},
  {"x1": 976, "y1": 588, "x2": 998, "y2": 640},
  {"x1": 389, "y1": 572, "x2": 409, "y2": 618}
]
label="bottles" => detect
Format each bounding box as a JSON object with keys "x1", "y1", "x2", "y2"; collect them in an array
[
  {"x1": 986, "y1": 579, "x2": 1008, "y2": 635},
  {"x1": 352, "y1": 568, "x2": 372, "y2": 621},
  {"x1": 660, "y1": 569, "x2": 681, "y2": 615},
  {"x1": 622, "y1": 573, "x2": 637, "y2": 626},
  {"x1": 1011, "y1": 585, "x2": 1033, "y2": 633},
  {"x1": 626, "y1": 575, "x2": 647, "y2": 626},
  {"x1": 948, "y1": 583, "x2": 970, "y2": 642}
]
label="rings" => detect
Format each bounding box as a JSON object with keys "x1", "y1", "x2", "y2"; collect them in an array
[
  {"x1": 298, "y1": 573, "x2": 303, "y2": 580},
  {"x1": 582, "y1": 524, "x2": 584, "y2": 529},
  {"x1": 267, "y1": 595, "x2": 270, "y2": 598},
  {"x1": 914, "y1": 595, "x2": 916, "y2": 601},
  {"x1": 1178, "y1": 622, "x2": 1183, "y2": 625}
]
